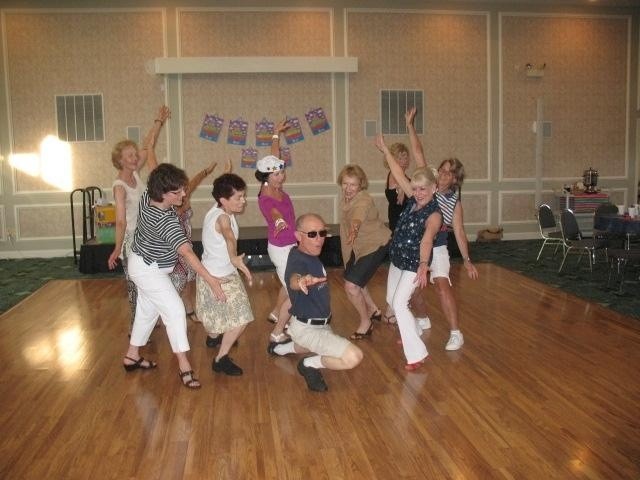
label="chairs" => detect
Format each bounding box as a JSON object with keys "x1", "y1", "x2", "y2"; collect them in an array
[{"x1": 530, "y1": 192, "x2": 639, "y2": 298}]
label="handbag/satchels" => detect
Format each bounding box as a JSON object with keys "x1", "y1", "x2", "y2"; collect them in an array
[{"x1": 475, "y1": 226, "x2": 505, "y2": 243}]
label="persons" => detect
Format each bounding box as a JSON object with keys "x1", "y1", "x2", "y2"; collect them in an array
[
  {"x1": 195, "y1": 157, "x2": 254, "y2": 374},
  {"x1": 385, "y1": 143, "x2": 412, "y2": 232},
  {"x1": 121, "y1": 128, "x2": 229, "y2": 390},
  {"x1": 404, "y1": 108, "x2": 479, "y2": 353},
  {"x1": 337, "y1": 163, "x2": 395, "y2": 342},
  {"x1": 108, "y1": 106, "x2": 171, "y2": 344},
  {"x1": 142, "y1": 132, "x2": 218, "y2": 328},
  {"x1": 375, "y1": 132, "x2": 444, "y2": 370},
  {"x1": 265, "y1": 213, "x2": 363, "y2": 392},
  {"x1": 254, "y1": 113, "x2": 298, "y2": 343}
]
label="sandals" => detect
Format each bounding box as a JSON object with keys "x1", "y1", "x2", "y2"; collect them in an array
[
  {"x1": 184, "y1": 309, "x2": 203, "y2": 323},
  {"x1": 178, "y1": 365, "x2": 201, "y2": 389},
  {"x1": 121, "y1": 354, "x2": 158, "y2": 370},
  {"x1": 348, "y1": 306, "x2": 399, "y2": 341},
  {"x1": 267, "y1": 311, "x2": 290, "y2": 343}
]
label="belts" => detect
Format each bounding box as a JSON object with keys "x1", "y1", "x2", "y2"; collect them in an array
[{"x1": 293, "y1": 313, "x2": 334, "y2": 327}]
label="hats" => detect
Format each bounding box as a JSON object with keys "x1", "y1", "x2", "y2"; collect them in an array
[{"x1": 255, "y1": 154, "x2": 286, "y2": 173}]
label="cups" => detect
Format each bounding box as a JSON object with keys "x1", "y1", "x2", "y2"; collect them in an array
[
  {"x1": 570, "y1": 184, "x2": 576, "y2": 194},
  {"x1": 628, "y1": 207, "x2": 636, "y2": 218},
  {"x1": 616, "y1": 206, "x2": 625, "y2": 216},
  {"x1": 631, "y1": 204, "x2": 639, "y2": 214},
  {"x1": 564, "y1": 184, "x2": 570, "y2": 195}
]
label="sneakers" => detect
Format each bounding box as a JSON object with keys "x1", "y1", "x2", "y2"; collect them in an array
[
  {"x1": 268, "y1": 339, "x2": 296, "y2": 357},
  {"x1": 413, "y1": 315, "x2": 432, "y2": 330},
  {"x1": 297, "y1": 357, "x2": 330, "y2": 392},
  {"x1": 444, "y1": 329, "x2": 464, "y2": 350}
]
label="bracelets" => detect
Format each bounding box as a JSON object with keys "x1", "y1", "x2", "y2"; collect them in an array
[
  {"x1": 274, "y1": 218, "x2": 287, "y2": 227},
  {"x1": 155, "y1": 119, "x2": 165, "y2": 125},
  {"x1": 463, "y1": 257, "x2": 471, "y2": 264},
  {"x1": 271, "y1": 134, "x2": 279, "y2": 138},
  {"x1": 203, "y1": 169, "x2": 208, "y2": 177},
  {"x1": 419, "y1": 261, "x2": 430, "y2": 266}
]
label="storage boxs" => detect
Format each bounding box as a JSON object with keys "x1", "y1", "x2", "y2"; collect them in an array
[
  {"x1": 97, "y1": 221, "x2": 115, "y2": 244},
  {"x1": 92, "y1": 203, "x2": 118, "y2": 224}
]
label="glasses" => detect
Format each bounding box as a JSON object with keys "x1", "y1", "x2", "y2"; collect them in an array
[{"x1": 297, "y1": 228, "x2": 327, "y2": 238}]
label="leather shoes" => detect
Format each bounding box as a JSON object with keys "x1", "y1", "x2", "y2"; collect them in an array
[
  {"x1": 205, "y1": 332, "x2": 240, "y2": 347},
  {"x1": 211, "y1": 353, "x2": 244, "y2": 376}
]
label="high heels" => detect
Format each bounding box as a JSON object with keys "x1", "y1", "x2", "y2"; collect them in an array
[{"x1": 403, "y1": 352, "x2": 434, "y2": 371}]
